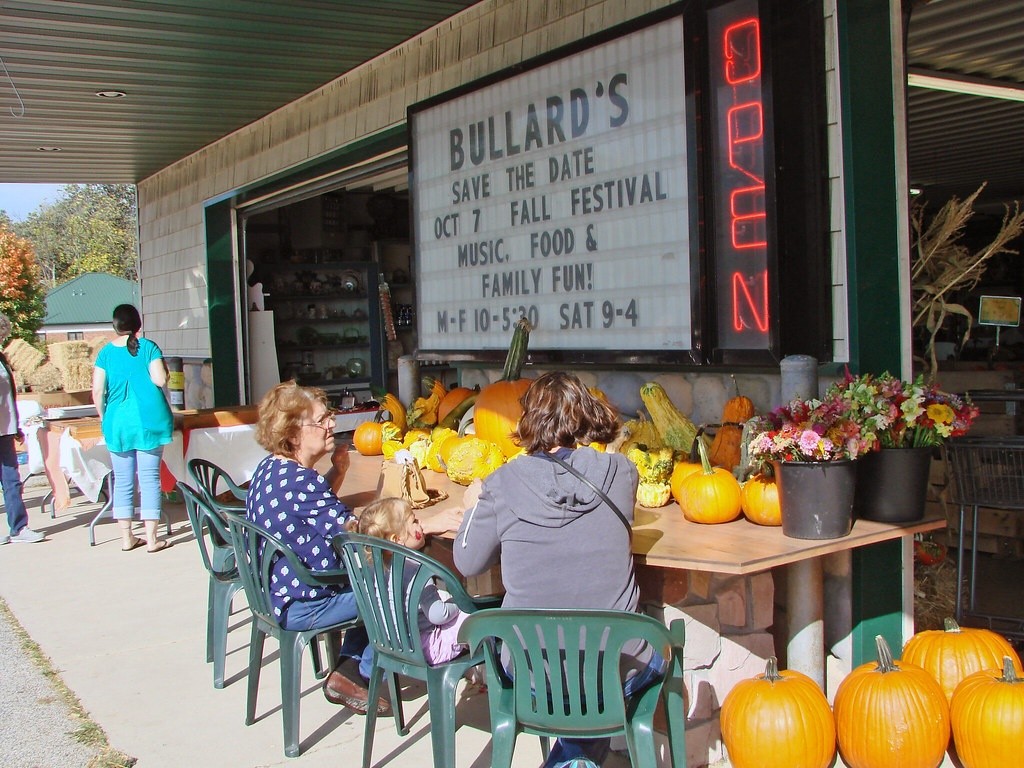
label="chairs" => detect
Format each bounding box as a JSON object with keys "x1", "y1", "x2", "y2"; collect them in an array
[
  {"x1": 455, "y1": 607, "x2": 689, "y2": 768},
  {"x1": 176, "y1": 480, "x2": 262, "y2": 690},
  {"x1": 219, "y1": 509, "x2": 366, "y2": 757},
  {"x1": 186, "y1": 459, "x2": 250, "y2": 614},
  {"x1": 332, "y1": 533, "x2": 507, "y2": 768}
]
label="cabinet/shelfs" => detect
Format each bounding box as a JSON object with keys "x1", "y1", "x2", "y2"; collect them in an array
[{"x1": 257, "y1": 260, "x2": 381, "y2": 394}]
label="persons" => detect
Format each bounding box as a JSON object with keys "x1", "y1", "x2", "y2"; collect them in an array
[
  {"x1": 0, "y1": 312, "x2": 47, "y2": 545},
  {"x1": 92, "y1": 305, "x2": 175, "y2": 553},
  {"x1": 239, "y1": 378, "x2": 390, "y2": 716},
  {"x1": 453, "y1": 368, "x2": 668, "y2": 768},
  {"x1": 345, "y1": 498, "x2": 494, "y2": 692}
]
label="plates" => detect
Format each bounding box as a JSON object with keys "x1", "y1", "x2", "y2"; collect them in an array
[{"x1": 346, "y1": 357, "x2": 365, "y2": 376}]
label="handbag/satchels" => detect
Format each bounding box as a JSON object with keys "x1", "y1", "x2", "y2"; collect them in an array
[{"x1": 375, "y1": 453, "x2": 450, "y2": 509}]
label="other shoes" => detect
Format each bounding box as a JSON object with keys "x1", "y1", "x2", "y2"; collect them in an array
[
  {"x1": 545, "y1": 744, "x2": 601, "y2": 768},
  {"x1": 320, "y1": 669, "x2": 390, "y2": 716}
]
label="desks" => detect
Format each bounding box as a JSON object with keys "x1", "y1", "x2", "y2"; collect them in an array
[
  {"x1": 39, "y1": 405, "x2": 389, "y2": 547},
  {"x1": 328, "y1": 451, "x2": 947, "y2": 768}
]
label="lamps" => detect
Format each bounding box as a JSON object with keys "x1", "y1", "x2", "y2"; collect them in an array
[{"x1": 905, "y1": 69, "x2": 1024, "y2": 102}]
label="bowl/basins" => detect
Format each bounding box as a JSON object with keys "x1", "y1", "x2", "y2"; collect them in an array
[
  {"x1": 319, "y1": 332, "x2": 337, "y2": 345},
  {"x1": 298, "y1": 373, "x2": 321, "y2": 380}
]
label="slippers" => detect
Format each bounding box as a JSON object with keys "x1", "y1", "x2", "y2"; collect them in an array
[
  {"x1": 147, "y1": 539, "x2": 174, "y2": 553},
  {"x1": 121, "y1": 538, "x2": 145, "y2": 552}
]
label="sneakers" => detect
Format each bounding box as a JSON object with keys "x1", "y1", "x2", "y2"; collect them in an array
[
  {"x1": 9, "y1": 526, "x2": 45, "y2": 543},
  {"x1": 0, "y1": 535, "x2": 10, "y2": 544}
]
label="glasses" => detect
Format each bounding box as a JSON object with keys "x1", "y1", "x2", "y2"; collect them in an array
[{"x1": 301, "y1": 405, "x2": 337, "y2": 432}]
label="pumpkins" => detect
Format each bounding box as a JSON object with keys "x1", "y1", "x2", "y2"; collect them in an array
[
  {"x1": 607, "y1": 375, "x2": 783, "y2": 527},
  {"x1": 720, "y1": 617, "x2": 1024, "y2": 768},
  {"x1": 354, "y1": 316, "x2": 607, "y2": 486}
]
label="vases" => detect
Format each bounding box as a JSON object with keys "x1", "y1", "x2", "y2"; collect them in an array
[
  {"x1": 774, "y1": 458, "x2": 855, "y2": 539},
  {"x1": 855, "y1": 445, "x2": 930, "y2": 523}
]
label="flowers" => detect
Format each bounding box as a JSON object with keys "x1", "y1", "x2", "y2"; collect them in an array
[
  {"x1": 827, "y1": 363, "x2": 978, "y2": 446},
  {"x1": 741, "y1": 397, "x2": 881, "y2": 460}
]
